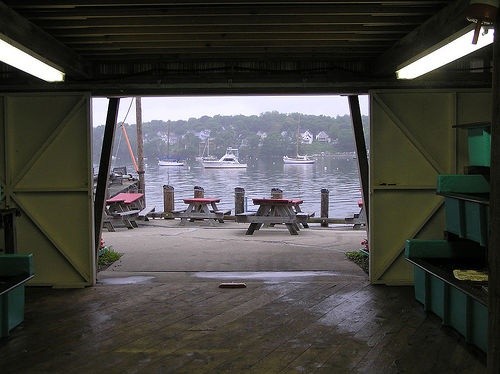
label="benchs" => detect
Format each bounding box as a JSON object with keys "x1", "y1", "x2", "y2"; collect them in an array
[
  {"x1": 161, "y1": 210, "x2": 186, "y2": 219},
  {"x1": 138, "y1": 206, "x2": 155, "y2": 222},
  {"x1": 345, "y1": 217, "x2": 354, "y2": 224},
  {"x1": 295, "y1": 211, "x2": 315, "y2": 223},
  {"x1": 235, "y1": 212, "x2": 257, "y2": 223},
  {"x1": 112, "y1": 209, "x2": 140, "y2": 220},
  {"x1": 214, "y1": 209, "x2": 231, "y2": 219}
]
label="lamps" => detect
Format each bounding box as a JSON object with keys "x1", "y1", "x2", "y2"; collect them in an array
[
  {"x1": 393, "y1": 21, "x2": 495, "y2": 80},
  {"x1": 0, "y1": 33, "x2": 66, "y2": 83}
]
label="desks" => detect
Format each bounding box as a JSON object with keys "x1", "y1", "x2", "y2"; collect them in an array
[
  {"x1": 246, "y1": 198, "x2": 304, "y2": 235},
  {"x1": 353, "y1": 200, "x2": 365, "y2": 230},
  {"x1": 179, "y1": 197, "x2": 221, "y2": 227},
  {"x1": 106, "y1": 193, "x2": 144, "y2": 232}
]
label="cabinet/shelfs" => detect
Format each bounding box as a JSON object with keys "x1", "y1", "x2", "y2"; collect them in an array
[
  {"x1": 0, "y1": 252, "x2": 35, "y2": 338},
  {"x1": 403, "y1": 120, "x2": 491, "y2": 355}
]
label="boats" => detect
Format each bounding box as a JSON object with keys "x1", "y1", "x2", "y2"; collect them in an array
[
  {"x1": 158, "y1": 158, "x2": 187, "y2": 166},
  {"x1": 201, "y1": 147, "x2": 248, "y2": 168},
  {"x1": 283, "y1": 153, "x2": 315, "y2": 164}
]
